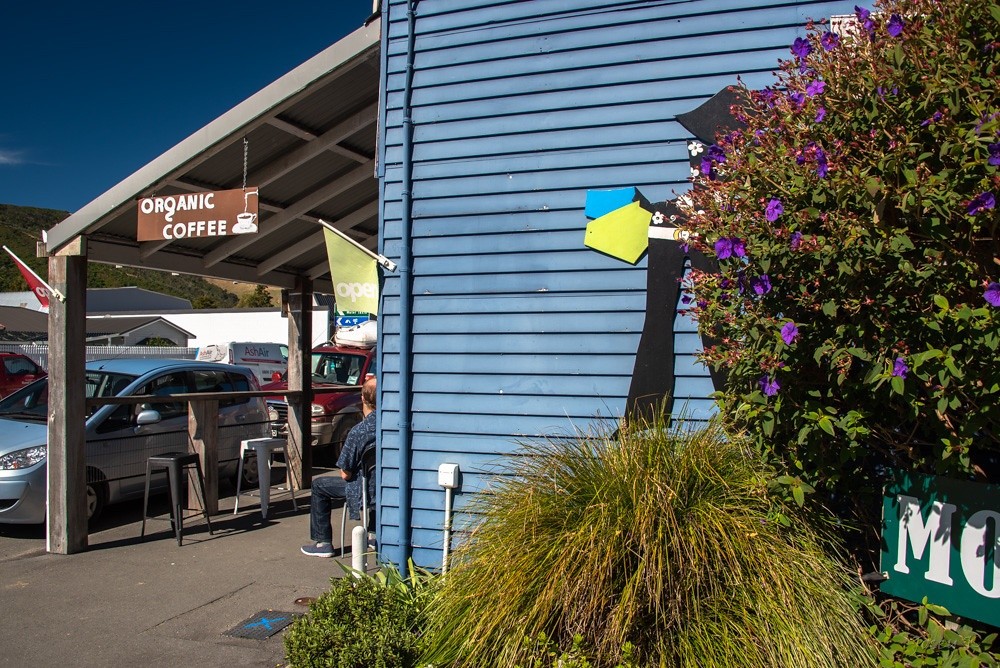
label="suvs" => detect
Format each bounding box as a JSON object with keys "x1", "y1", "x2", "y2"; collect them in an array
[
  {"x1": 260, "y1": 340, "x2": 377, "y2": 462},
  {"x1": 0, "y1": 357, "x2": 271, "y2": 535}
]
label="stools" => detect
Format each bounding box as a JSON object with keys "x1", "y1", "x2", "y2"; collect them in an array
[
  {"x1": 233, "y1": 437, "x2": 298, "y2": 520},
  {"x1": 140, "y1": 452, "x2": 214, "y2": 546}
]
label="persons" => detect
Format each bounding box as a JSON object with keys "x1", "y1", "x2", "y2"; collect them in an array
[
  {"x1": 341, "y1": 356, "x2": 360, "y2": 377},
  {"x1": 301, "y1": 377, "x2": 376, "y2": 558}
]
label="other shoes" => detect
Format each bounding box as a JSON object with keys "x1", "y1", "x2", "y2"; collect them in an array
[{"x1": 301, "y1": 542, "x2": 336, "y2": 558}]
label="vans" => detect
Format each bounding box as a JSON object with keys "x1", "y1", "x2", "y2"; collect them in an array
[{"x1": 0, "y1": 350, "x2": 49, "y2": 407}]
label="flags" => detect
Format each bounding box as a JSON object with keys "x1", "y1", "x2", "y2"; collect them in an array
[
  {"x1": 9, "y1": 255, "x2": 50, "y2": 307},
  {"x1": 323, "y1": 226, "x2": 380, "y2": 316}
]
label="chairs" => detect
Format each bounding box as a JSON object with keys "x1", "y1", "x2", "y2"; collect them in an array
[
  {"x1": 327, "y1": 367, "x2": 348, "y2": 383},
  {"x1": 340, "y1": 448, "x2": 375, "y2": 558},
  {"x1": 111, "y1": 379, "x2": 132, "y2": 424}
]
